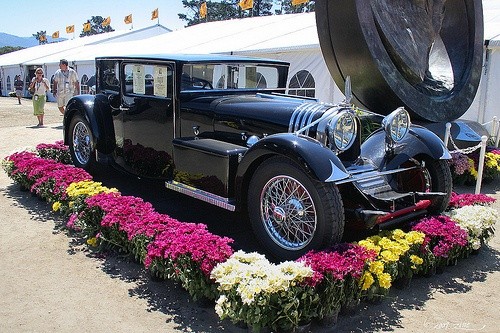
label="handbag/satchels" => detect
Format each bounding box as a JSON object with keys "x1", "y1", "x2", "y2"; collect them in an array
[{"x1": 29, "y1": 78, "x2": 36, "y2": 95}]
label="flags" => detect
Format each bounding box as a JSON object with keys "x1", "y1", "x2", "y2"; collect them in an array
[
  {"x1": 151, "y1": 9, "x2": 158, "y2": 20},
  {"x1": 66, "y1": 25, "x2": 75, "y2": 34},
  {"x1": 83, "y1": 22, "x2": 91, "y2": 32},
  {"x1": 102, "y1": 17, "x2": 111, "y2": 27},
  {"x1": 291, "y1": 0, "x2": 309, "y2": 6},
  {"x1": 238, "y1": 0, "x2": 253, "y2": 10},
  {"x1": 51, "y1": 31, "x2": 59, "y2": 38},
  {"x1": 124, "y1": 15, "x2": 132, "y2": 24},
  {"x1": 199, "y1": 3, "x2": 207, "y2": 18},
  {"x1": 39, "y1": 32, "x2": 46, "y2": 41}
]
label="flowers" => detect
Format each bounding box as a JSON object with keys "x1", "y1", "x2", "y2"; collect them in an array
[
  {"x1": 449, "y1": 148, "x2": 500, "y2": 180},
  {"x1": 121, "y1": 138, "x2": 172, "y2": 176},
  {"x1": 174, "y1": 170, "x2": 229, "y2": 195},
  {"x1": 68, "y1": 193, "x2": 234, "y2": 300},
  {"x1": 209, "y1": 190, "x2": 499, "y2": 332},
  {"x1": 2, "y1": 140, "x2": 121, "y2": 217}
]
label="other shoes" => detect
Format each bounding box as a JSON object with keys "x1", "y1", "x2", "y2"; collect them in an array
[{"x1": 37, "y1": 124, "x2": 43, "y2": 127}]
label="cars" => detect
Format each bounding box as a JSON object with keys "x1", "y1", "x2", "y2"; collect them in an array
[
  {"x1": 115, "y1": 68, "x2": 213, "y2": 97},
  {"x1": 61, "y1": 55, "x2": 454, "y2": 267}
]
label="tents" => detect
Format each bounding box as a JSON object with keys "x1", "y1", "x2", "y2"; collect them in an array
[{"x1": 0, "y1": 0, "x2": 500, "y2": 145}]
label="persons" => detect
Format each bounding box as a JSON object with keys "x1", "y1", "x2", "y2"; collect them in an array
[
  {"x1": 29, "y1": 68, "x2": 51, "y2": 126},
  {"x1": 14, "y1": 75, "x2": 24, "y2": 104},
  {"x1": 52, "y1": 59, "x2": 80, "y2": 115}
]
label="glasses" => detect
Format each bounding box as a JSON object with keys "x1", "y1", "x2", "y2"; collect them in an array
[{"x1": 36, "y1": 73, "x2": 42, "y2": 74}]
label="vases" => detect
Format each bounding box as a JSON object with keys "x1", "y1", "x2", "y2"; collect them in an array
[
  {"x1": 38, "y1": 196, "x2": 46, "y2": 201},
  {"x1": 195, "y1": 295, "x2": 213, "y2": 308},
  {"x1": 31, "y1": 189, "x2": 37, "y2": 197},
  {"x1": 296, "y1": 319, "x2": 312, "y2": 333},
  {"x1": 398, "y1": 274, "x2": 412, "y2": 290},
  {"x1": 318, "y1": 309, "x2": 338, "y2": 329},
  {"x1": 482, "y1": 170, "x2": 494, "y2": 184},
  {"x1": 342, "y1": 304, "x2": 358, "y2": 317},
  {"x1": 370, "y1": 297, "x2": 383, "y2": 305},
  {"x1": 20, "y1": 183, "x2": 28, "y2": 191},
  {"x1": 423, "y1": 262, "x2": 436, "y2": 278},
  {"x1": 465, "y1": 173, "x2": 475, "y2": 186},
  {"x1": 247, "y1": 323, "x2": 271, "y2": 333}
]
